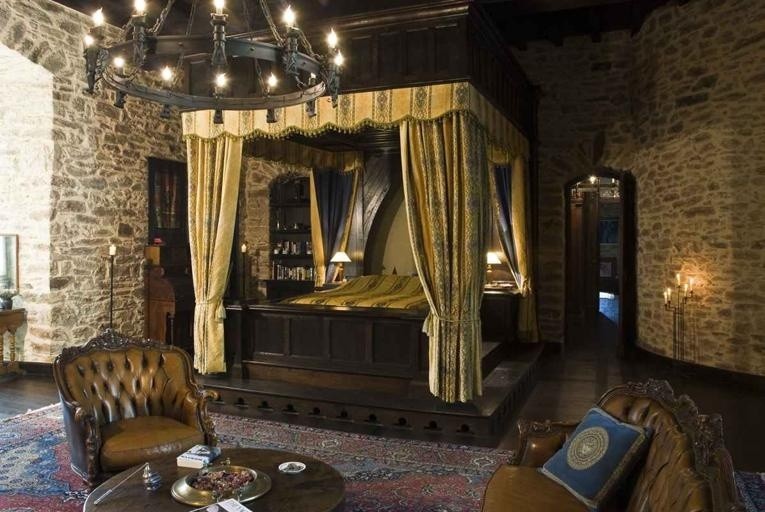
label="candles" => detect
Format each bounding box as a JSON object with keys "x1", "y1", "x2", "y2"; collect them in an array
[
  {"x1": 663, "y1": 273, "x2": 695, "y2": 305},
  {"x1": 109, "y1": 244, "x2": 116, "y2": 254},
  {"x1": 242, "y1": 243, "x2": 247, "y2": 252}
]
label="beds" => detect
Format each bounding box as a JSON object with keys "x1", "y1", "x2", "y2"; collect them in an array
[{"x1": 182, "y1": 1, "x2": 544, "y2": 448}]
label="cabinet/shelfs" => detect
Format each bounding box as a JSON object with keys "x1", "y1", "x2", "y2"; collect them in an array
[
  {"x1": 144, "y1": 157, "x2": 239, "y2": 359},
  {"x1": 263, "y1": 170, "x2": 314, "y2": 297},
  {"x1": 0, "y1": 308, "x2": 26, "y2": 376}
]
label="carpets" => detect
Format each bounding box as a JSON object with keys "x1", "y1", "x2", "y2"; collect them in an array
[{"x1": 1, "y1": 400, "x2": 765, "y2": 512}]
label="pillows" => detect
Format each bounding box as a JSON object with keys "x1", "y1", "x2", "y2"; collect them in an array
[{"x1": 536, "y1": 404, "x2": 652, "y2": 510}]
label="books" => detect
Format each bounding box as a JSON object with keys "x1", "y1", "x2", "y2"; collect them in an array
[
  {"x1": 175, "y1": 444, "x2": 221, "y2": 470},
  {"x1": 269, "y1": 238, "x2": 316, "y2": 282},
  {"x1": 187, "y1": 497, "x2": 254, "y2": 512}
]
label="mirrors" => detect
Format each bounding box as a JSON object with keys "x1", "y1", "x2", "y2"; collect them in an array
[
  {"x1": 0, "y1": 234, "x2": 17, "y2": 295},
  {"x1": 563, "y1": 165, "x2": 636, "y2": 361}
]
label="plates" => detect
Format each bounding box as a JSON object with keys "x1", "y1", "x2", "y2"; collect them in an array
[{"x1": 278, "y1": 461, "x2": 307, "y2": 473}]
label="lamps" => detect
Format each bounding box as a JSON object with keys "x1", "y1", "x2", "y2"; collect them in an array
[
  {"x1": 83, "y1": 0, "x2": 348, "y2": 126},
  {"x1": 486, "y1": 251, "x2": 502, "y2": 284},
  {"x1": 330, "y1": 252, "x2": 352, "y2": 282}
]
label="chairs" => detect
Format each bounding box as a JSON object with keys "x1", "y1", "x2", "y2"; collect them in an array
[
  {"x1": 53, "y1": 328, "x2": 219, "y2": 488},
  {"x1": 481, "y1": 377, "x2": 747, "y2": 512}
]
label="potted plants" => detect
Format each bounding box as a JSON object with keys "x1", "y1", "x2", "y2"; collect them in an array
[{"x1": 0, "y1": 292, "x2": 14, "y2": 309}]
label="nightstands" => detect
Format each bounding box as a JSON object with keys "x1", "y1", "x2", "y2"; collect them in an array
[{"x1": 480, "y1": 288, "x2": 519, "y2": 341}]
